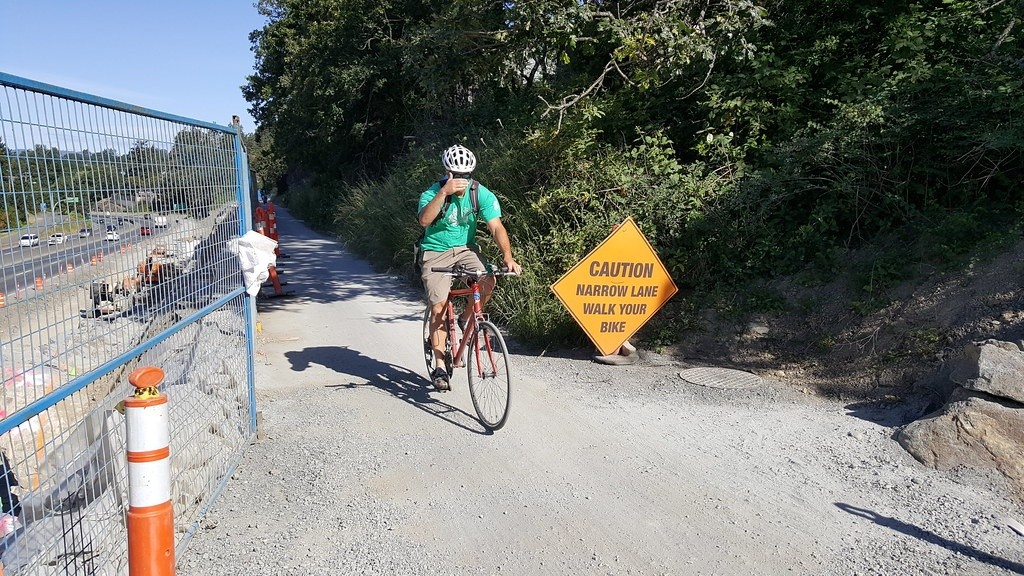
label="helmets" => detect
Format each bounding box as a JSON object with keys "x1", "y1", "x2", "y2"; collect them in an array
[{"x1": 442, "y1": 144, "x2": 476, "y2": 173}]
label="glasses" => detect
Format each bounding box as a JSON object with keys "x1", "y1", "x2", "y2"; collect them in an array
[{"x1": 453, "y1": 173, "x2": 471, "y2": 179}]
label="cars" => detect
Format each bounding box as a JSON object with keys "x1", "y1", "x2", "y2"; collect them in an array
[
  {"x1": 116, "y1": 218, "x2": 135, "y2": 226},
  {"x1": 144, "y1": 213, "x2": 151, "y2": 219},
  {"x1": 47, "y1": 233, "x2": 69, "y2": 246},
  {"x1": 106, "y1": 224, "x2": 116, "y2": 233},
  {"x1": 97, "y1": 218, "x2": 105, "y2": 223},
  {"x1": 154, "y1": 216, "x2": 168, "y2": 228},
  {"x1": 18, "y1": 234, "x2": 38, "y2": 247},
  {"x1": 78, "y1": 228, "x2": 92, "y2": 238},
  {"x1": 105, "y1": 232, "x2": 120, "y2": 242},
  {"x1": 141, "y1": 226, "x2": 152, "y2": 235}
]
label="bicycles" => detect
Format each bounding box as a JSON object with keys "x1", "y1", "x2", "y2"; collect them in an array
[
  {"x1": 422, "y1": 260, "x2": 517, "y2": 430},
  {"x1": 261, "y1": 192, "x2": 267, "y2": 204}
]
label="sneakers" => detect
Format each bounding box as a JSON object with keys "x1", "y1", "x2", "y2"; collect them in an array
[
  {"x1": 433, "y1": 368, "x2": 449, "y2": 390},
  {"x1": 458, "y1": 313, "x2": 472, "y2": 344}
]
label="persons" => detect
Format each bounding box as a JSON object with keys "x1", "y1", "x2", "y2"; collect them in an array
[
  {"x1": 260, "y1": 185, "x2": 268, "y2": 204},
  {"x1": 418, "y1": 144, "x2": 523, "y2": 389}
]
